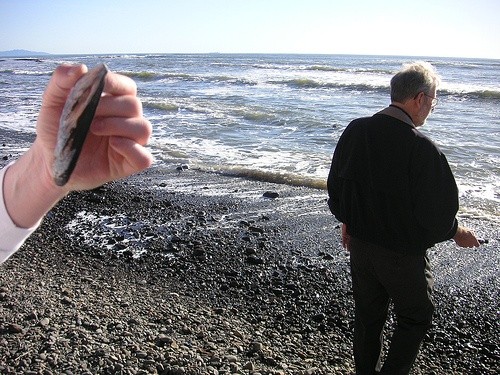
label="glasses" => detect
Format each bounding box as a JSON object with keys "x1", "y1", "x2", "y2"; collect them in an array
[{"x1": 413, "y1": 91, "x2": 438, "y2": 107}]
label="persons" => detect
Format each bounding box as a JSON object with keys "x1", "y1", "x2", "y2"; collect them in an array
[
  {"x1": 0, "y1": 61, "x2": 153, "y2": 265},
  {"x1": 327, "y1": 60, "x2": 481, "y2": 375}
]
